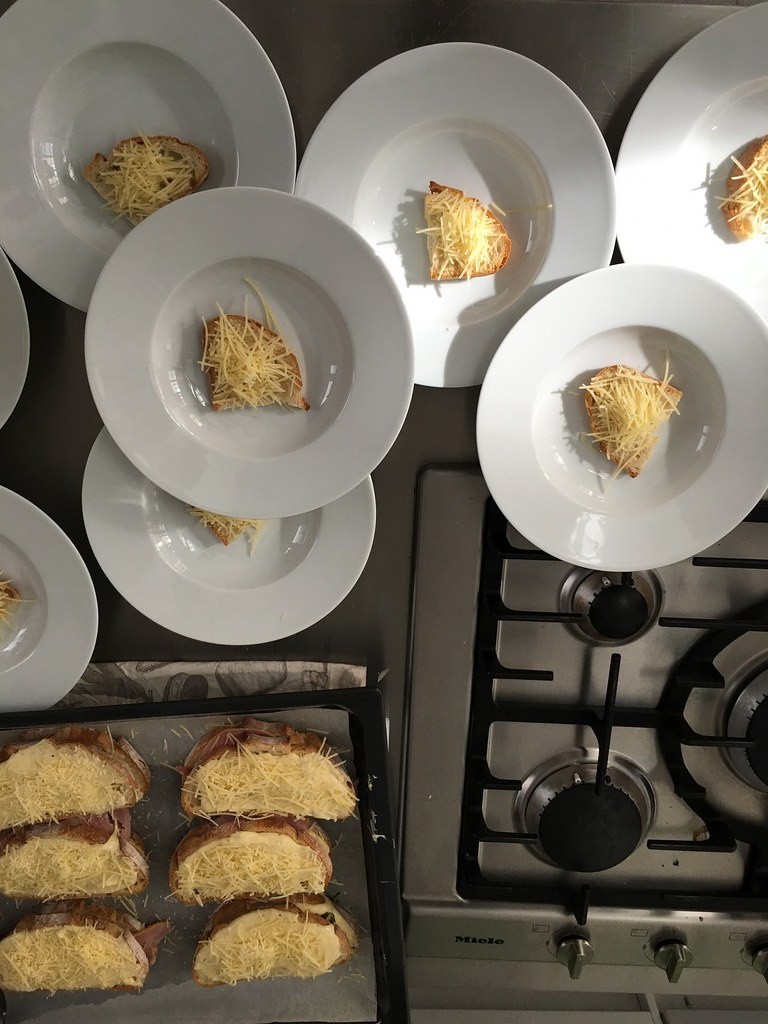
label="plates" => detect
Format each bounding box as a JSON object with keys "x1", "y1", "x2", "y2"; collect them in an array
[
  {"x1": 1, "y1": 1, "x2": 767, "y2": 521},
  {"x1": 82, "y1": 424, "x2": 377, "y2": 645},
  {"x1": 1, "y1": 487, "x2": 97, "y2": 713},
  {"x1": 475, "y1": 263, "x2": 768, "y2": 571}
]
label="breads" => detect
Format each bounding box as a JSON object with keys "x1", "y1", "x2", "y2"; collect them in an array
[
  {"x1": 423, "y1": 181, "x2": 512, "y2": 280},
  {"x1": 192, "y1": 507, "x2": 266, "y2": 546},
  {"x1": 0, "y1": 581, "x2": 20, "y2": 615},
  {"x1": 0, "y1": 713, "x2": 355, "y2": 991},
  {"x1": 202, "y1": 313, "x2": 311, "y2": 412},
  {"x1": 724, "y1": 133, "x2": 768, "y2": 243},
  {"x1": 84, "y1": 135, "x2": 210, "y2": 225},
  {"x1": 584, "y1": 364, "x2": 683, "y2": 479}
]
